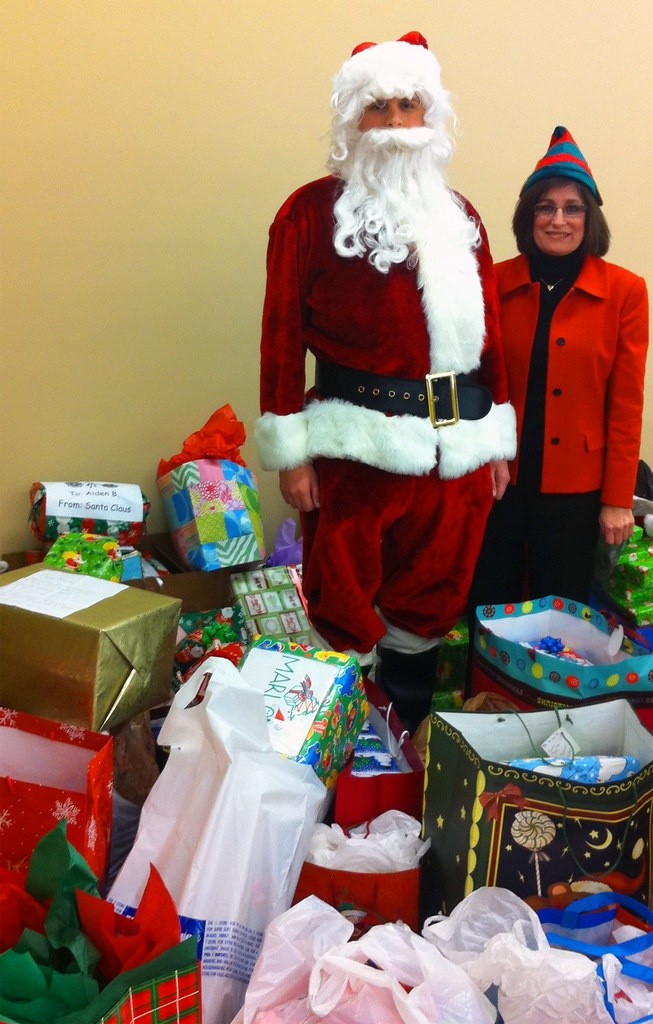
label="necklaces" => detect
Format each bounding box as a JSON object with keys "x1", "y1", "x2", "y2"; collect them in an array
[{"x1": 540, "y1": 277, "x2": 563, "y2": 291}]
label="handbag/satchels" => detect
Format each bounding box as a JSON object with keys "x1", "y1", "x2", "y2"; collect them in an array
[{"x1": 0, "y1": 457, "x2": 653, "y2": 1024}]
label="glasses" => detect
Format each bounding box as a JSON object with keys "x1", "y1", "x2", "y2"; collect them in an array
[{"x1": 534, "y1": 202, "x2": 588, "y2": 216}]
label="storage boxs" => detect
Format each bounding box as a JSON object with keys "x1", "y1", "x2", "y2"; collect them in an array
[
  {"x1": 236, "y1": 633, "x2": 371, "y2": 793},
  {"x1": 123, "y1": 531, "x2": 247, "y2": 614},
  {"x1": 28, "y1": 482, "x2": 150, "y2": 542},
  {"x1": 0, "y1": 560, "x2": 183, "y2": 735},
  {"x1": 602, "y1": 524, "x2": 653, "y2": 628},
  {"x1": 225, "y1": 564, "x2": 312, "y2": 649}
]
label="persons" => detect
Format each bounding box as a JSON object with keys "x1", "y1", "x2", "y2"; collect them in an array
[
  {"x1": 253, "y1": 32, "x2": 518, "y2": 740},
  {"x1": 492, "y1": 127, "x2": 650, "y2": 605}
]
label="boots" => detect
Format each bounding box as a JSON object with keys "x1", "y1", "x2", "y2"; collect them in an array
[
  {"x1": 308, "y1": 626, "x2": 374, "y2": 678},
  {"x1": 375, "y1": 605, "x2": 439, "y2": 740}
]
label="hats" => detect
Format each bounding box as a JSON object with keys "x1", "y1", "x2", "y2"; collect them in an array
[
  {"x1": 520, "y1": 126, "x2": 604, "y2": 207},
  {"x1": 331, "y1": 30, "x2": 442, "y2": 91}
]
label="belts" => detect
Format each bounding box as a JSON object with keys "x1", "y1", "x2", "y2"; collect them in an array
[{"x1": 314, "y1": 358, "x2": 492, "y2": 427}]
